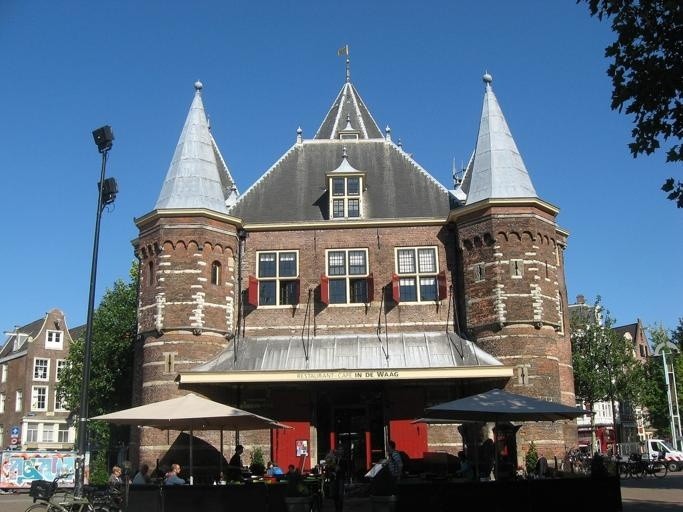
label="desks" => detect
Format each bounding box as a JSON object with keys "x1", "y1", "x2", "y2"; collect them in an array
[{"x1": 68, "y1": 120, "x2": 119, "y2": 495}]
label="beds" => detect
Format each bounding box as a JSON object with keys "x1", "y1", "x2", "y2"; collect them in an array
[
  {"x1": 423, "y1": 388, "x2": 596, "y2": 482},
  {"x1": 84, "y1": 392, "x2": 295, "y2": 484},
  {"x1": 407, "y1": 416, "x2": 480, "y2": 426}
]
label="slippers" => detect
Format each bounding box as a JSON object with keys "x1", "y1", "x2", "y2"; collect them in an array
[{"x1": 399, "y1": 451, "x2": 409, "y2": 471}]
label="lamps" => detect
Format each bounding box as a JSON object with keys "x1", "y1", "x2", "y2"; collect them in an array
[{"x1": 212, "y1": 462, "x2": 336, "y2": 512}]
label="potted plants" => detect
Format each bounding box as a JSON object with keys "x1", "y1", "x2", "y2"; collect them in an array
[{"x1": 294, "y1": 438, "x2": 309, "y2": 458}]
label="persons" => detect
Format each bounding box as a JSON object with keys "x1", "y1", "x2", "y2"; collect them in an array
[
  {"x1": 478, "y1": 437, "x2": 498, "y2": 482},
  {"x1": 133, "y1": 463, "x2": 149, "y2": 484},
  {"x1": 227, "y1": 444, "x2": 246, "y2": 482},
  {"x1": 108, "y1": 465, "x2": 124, "y2": 485},
  {"x1": 331, "y1": 443, "x2": 346, "y2": 512},
  {"x1": 265, "y1": 461, "x2": 297, "y2": 476},
  {"x1": 163, "y1": 463, "x2": 186, "y2": 484},
  {"x1": 386, "y1": 440, "x2": 403, "y2": 500},
  {"x1": 213, "y1": 470, "x2": 228, "y2": 485}
]
